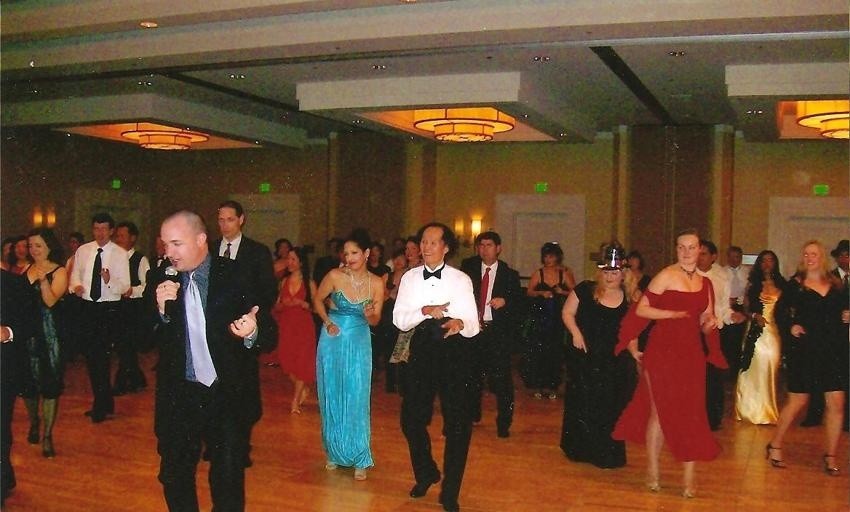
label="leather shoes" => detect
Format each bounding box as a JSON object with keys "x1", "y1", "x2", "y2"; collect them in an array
[{"x1": 409, "y1": 470, "x2": 442, "y2": 498}]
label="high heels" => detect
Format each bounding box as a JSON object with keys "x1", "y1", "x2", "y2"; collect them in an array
[
  {"x1": 764, "y1": 442, "x2": 788, "y2": 469},
  {"x1": 822, "y1": 453, "x2": 842, "y2": 477}
]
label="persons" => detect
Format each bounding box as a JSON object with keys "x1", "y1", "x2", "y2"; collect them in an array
[
  {"x1": 609, "y1": 227, "x2": 730, "y2": 499},
  {"x1": 764, "y1": 240, "x2": 850, "y2": 478},
  {"x1": 802, "y1": 240, "x2": 850, "y2": 425},
  {"x1": 695, "y1": 241, "x2": 789, "y2": 430},
  {"x1": 391, "y1": 222, "x2": 482, "y2": 512},
  {"x1": 210, "y1": 201, "x2": 344, "y2": 413},
  {"x1": 130, "y1": 207, "x2": 279, "y2": 512},
  {"x1": 366, "y1": 236, "x2": 423, "y2": 391},
  {"x1": 1, "y1": 262, "x2": 43, "y2": 494},
  {"x1": 459, "y1": 230, "x2": 655, "y2": 466},
  {"x1": 312, "y1": 226, "x2": 385, "y2": 482},
  {"x1": 0, "y1": 212, "x2": 171, "y2": 458}
]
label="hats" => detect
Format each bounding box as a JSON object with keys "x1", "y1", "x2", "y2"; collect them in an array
[
  {"x1": 596, "y1": 246, "x2": 633, "y2": 271},
  {"x1": 830, "y1": 240, "x2": 849, "y2": 257}
]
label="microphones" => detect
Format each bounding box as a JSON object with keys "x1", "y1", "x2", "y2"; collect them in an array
[{"x1": 165, "y1": 266, "x2": 178, "y2": 315}]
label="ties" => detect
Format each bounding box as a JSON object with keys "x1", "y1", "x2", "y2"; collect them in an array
[
  {"x1": 182, "y1": 269, "x2": 218, "y2": 387},
  {"x1": 89, "y1": 248, "x2": 103, "y2": 303},
  {"x1": 478, "y1": 267, "x2": 492, "y2": 331},
  {"x1": 222, "y1": 242, "x2": 232, "y2": 259}
]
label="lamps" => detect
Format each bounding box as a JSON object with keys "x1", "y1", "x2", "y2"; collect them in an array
[
  {"x1": 412, "y1": 108, "x2": 516, "y2": 143},
  {"x1": 119, "y1": 130, "x2": 209, "y2": 152},
  {"x1": 796, "y1": 99, "x2": 850, "y2": 141}
]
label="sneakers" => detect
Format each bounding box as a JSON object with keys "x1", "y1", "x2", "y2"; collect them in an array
[
  {"x1": 437, "y1": 492, "x2": 460, "y2": 512},
  {"x1": 473, "y1": 415, "x2": 483, "y2": 426},
  {"x1": 497, "y1": 430, "x2": 511, "y2": 440},
  {"x1": 84, "y1": 397, "x2": 115, "y2": 425},
  {"x1": 325, "y1": 462, "x2": 338, "y2": 471},
  {"x1": 646, "y1": 472, "x2": 664, "y2": 492},
  {"x1": 27, "y1": 416, "x2": 56, "y2": 460},
  {"x1": 113, "y1": 375, "x2": 149, "y2": 396},
  {"x1": 683, "y1": 484, "x2": 697, "y2": 499},
  {"x1": 532, "y1": 388, "x2": 561, "y2": 402},
  {"x1": 291, "y1": 386, "x2": 312, "y2": 419},
  {"x1": 354, "y1": 468, "x2": 368, "y2": 481}
]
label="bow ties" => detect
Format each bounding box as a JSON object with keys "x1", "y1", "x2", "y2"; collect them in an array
[{"x1": 423, "y1": 269, "x2": 442, "y2": 279}]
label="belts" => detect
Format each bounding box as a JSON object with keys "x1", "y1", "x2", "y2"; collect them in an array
[{"x1": 482, "y1": 321, "x2": 497, "y2": 331}]
